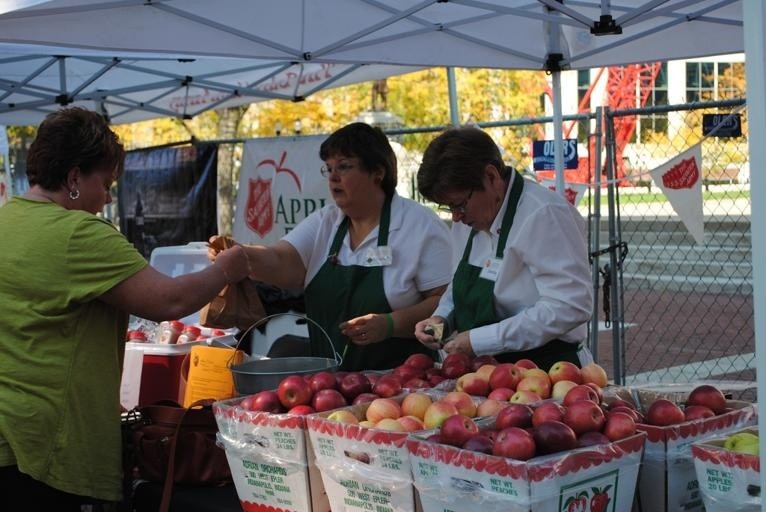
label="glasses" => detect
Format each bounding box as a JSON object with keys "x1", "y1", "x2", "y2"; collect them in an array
[
  {"x1": 437, "y1": 187, "x2": 475, "y2": 213},
  {"x1": 321, "y1": 158, "x2": 358, "y2": 178}
]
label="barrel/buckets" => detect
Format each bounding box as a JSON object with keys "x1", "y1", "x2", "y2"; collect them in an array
[{"x1": 228, "y1": 313, "x2": 343, "y2": 397}]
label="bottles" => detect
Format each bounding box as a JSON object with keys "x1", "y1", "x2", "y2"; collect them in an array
[{"x1": 127, "y1": 321, "x2": 225, "y2": 344}]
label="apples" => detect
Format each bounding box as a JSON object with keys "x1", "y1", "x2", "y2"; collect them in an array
[
  {"x1": 326, "y1": 391, "x2": 504, "y2": 433},
  {"x1": 239, "y1": 353, "x2": 497, "y2": 415},
  {"x1": 453, "y1": 359, "x2": 608, "y2": 405},
  {"x1": 604, "y1": 385, "x2": 726, "y2": 425},
  {"x1": 439, "y1": 384, "x2": 638, "y2": 461},
  {"x1": 723, "y1": 432, "x2": 760, "y2": 455}
]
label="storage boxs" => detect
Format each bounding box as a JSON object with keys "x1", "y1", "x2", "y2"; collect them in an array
[
  {"x1": 123, "y1": 322, "x2": 235, "y2": 408},
  {"x1": 418, "y1": 378, "x2": 618, "y2": 407},
  {"x1": 691, "y1": 427, "x2": 762, "y2": 512},
  {"x1": 601, "y1": 385, "x2": 756, "y2": 512},
  {"x1": 405, "y1": 410, "x2": 646, "y2": 512},
  {"x1": 212, "y1": 383, "x2": 408, "y2": 512},
  {"x1": 305, "y1": 390, "x2": 504, "y2": 512}
]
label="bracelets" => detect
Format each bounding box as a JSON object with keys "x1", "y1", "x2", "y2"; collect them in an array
[
  {"x1": 385, "y1": 312, "x2": 394, "y2": 340},
  {"x1": 214, "y1": 260, "x2": 228, "y2": 285}
]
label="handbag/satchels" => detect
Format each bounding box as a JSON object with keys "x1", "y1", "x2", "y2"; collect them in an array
[{"x1": 131, "y1": 398, "x2": 234, "y2": 488}]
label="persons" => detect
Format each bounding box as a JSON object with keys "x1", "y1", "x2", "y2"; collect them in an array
[
  {"x1": 207, "y1": 121, "x2": 455, "y2": 372},
  {"x1": 0, "y1": 106, "x2": 251, "y2": 511},
  {"x1": 415, "y1": 126, "x2": 595, "y2": 372}
]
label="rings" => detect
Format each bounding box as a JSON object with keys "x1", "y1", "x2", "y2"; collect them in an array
[{"x1": 361, "y1": 333, "x2": 367, "y2": 337}]
